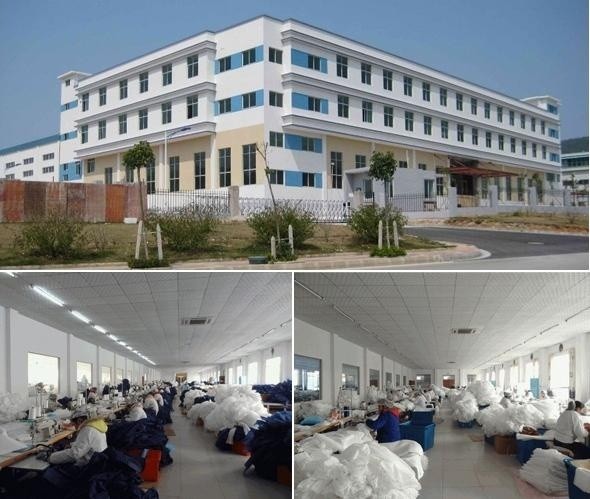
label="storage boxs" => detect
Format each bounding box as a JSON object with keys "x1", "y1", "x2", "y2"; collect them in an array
[
  {"x1": 563, "y1": 458, "x2": 590, "y2": 499},
  {"x1": 400, "y1": 423, "x2": 434, "y2": 452}
]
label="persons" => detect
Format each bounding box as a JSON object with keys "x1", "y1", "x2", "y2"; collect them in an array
[
  {"x1": 341, "y1": 382, "x2": 467, "y2": 421},
  {"x1": 366, "y1": 398, "x2": 402, "y2": 442},
  {"x1": 29, "y1": 374, "x2": 200, "y2": 484},
  {"x1": 498, "y1": 385, "x2": 590, "y2": 460}
]
